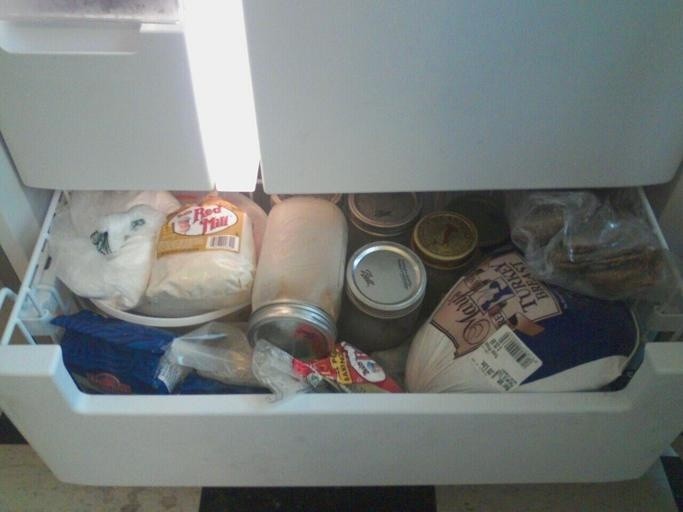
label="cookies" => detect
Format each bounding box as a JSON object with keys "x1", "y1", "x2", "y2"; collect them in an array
[{"x1": 508, "y1": 190, "x2": 663, "y2": 294}]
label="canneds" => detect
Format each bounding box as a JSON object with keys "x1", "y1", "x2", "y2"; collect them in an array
[
  {"x1": 408, "y1": 209, "x2": 479, "y2": 319},
  {"x1": 445, "y1": 200, "x2": 509, "y2": 261},
  {"x1": 342, "y1": 190, "x2": 423, "y2": 266},
  {"x1": 339, "y1": 239, "x2": 428, "y2": 355}
]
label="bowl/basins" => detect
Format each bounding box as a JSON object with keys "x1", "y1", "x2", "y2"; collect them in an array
[{"x1": 91, "y1": 192, "x2": 269, "y2": 329}]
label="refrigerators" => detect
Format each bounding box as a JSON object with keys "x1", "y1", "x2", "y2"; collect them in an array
[{"x1": 0, "y1": 2, "x2": 683, "y2": 512}]
